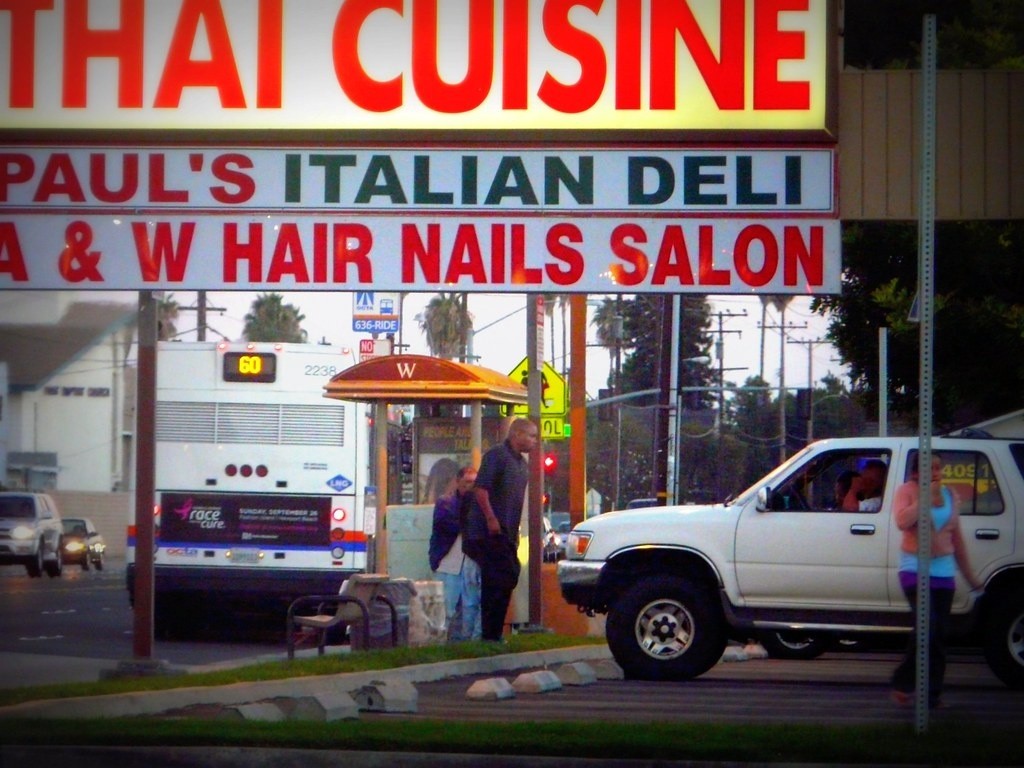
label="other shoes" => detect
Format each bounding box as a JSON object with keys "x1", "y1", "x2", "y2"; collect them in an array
[
  {"x1": 933, "y1": 693, "x2": 965, "y2": 711},
  {"x1": 892, "y1": 690, "x2": 913, "y2": 707}
]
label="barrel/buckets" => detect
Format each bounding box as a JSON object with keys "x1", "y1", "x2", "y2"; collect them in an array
[
  {"x1": 411, "y1": 581, "x2": 447, "y2": 647},
  {"x1": 343, "y1": 580, "x2": 411, "y2": 651}
]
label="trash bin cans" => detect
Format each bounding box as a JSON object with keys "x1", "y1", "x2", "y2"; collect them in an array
[{"x1": 350, "y1": 578, "x2": 418, "y2": 650}]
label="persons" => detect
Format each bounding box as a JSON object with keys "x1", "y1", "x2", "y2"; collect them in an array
[
  {"x1": 422, "y1": 458, "x2": 463, "y2": 503},
  {"x1": 462, "y1": 418, "x2": 539, "y2": 641},
  {"x1": 834, "y1": 470, "x2": 864, "y2": 505},
  {"x1": 429, "y1": 466, "x2": 482, "y2": 641},
  {"x1": 842, "y1": 459, "x2": 886, "y2": 513},
  {"x1": 895, "y1": 450, "x2": 986, "y2": 705}
]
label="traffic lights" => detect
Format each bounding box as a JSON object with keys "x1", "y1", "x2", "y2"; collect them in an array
[{"x1": 545, "y1": 454, "x2": 557, "y2": 473}]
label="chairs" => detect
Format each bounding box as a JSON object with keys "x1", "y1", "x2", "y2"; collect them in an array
[{"x1": 287, "y1": 573, "x2": 397, "y2": 659}]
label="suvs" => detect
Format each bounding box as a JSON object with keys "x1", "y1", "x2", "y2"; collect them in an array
[
  {"x1": 0, "y1": 491, "x2": 64, "y2": 579},
  {"x1": 555, "y1": 439, "x2": 1024, "y2": 694}
]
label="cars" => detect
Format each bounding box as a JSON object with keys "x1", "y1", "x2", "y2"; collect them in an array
[
  {"x1": 59, "y1": 515, "x2": 107, "y2": 573},
  {"x1": 542, "y1": 516, "x2": 572, "y2": 561}
]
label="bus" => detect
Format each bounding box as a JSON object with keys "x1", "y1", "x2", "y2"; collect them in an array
[{"x1": 126, "y1": 340, "x2": 382, "y2": 623}]
